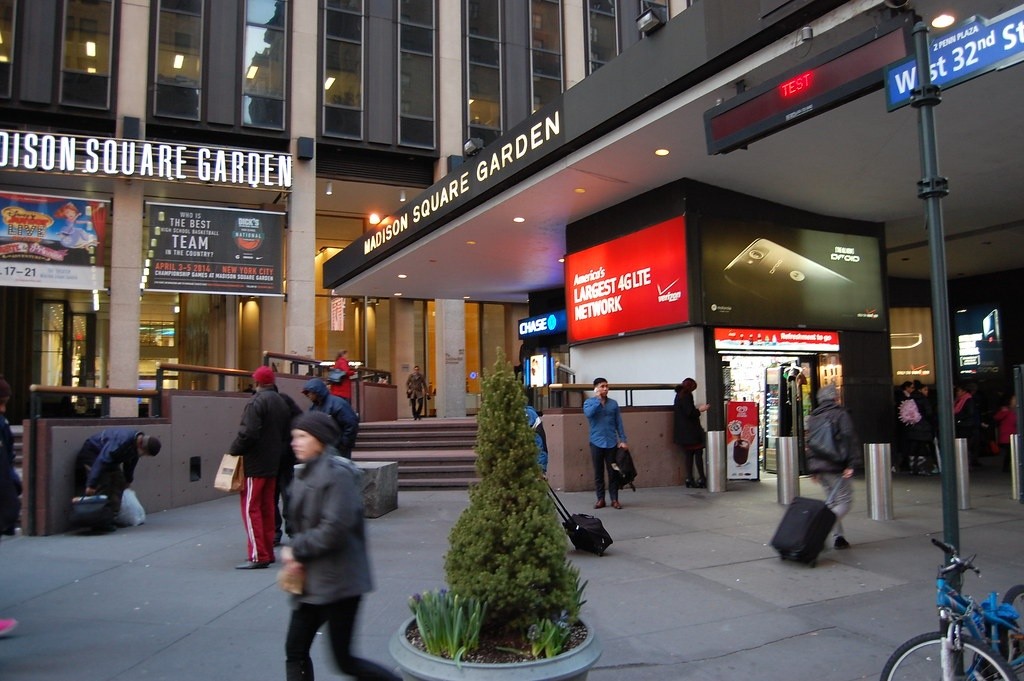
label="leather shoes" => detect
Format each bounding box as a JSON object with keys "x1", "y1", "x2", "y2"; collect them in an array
[
  {"x1": 611, "y1": 501, "x2": 622, "y2": 510},
  {"x1": 593, "y1": 497, "x2": 605, "y2": 509}
]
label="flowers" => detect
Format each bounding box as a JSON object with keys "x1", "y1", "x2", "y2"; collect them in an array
[{"x1": 407, "y1": 343, "x2": 592, "y2": 660}]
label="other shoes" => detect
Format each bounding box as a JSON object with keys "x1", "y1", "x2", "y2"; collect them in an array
[
  {"x1": 112, "y1": 515, "x2": 129, "y2": 528},
  {"x1": 235, "y1": 560, "x2": 269, "y2": 569},
  {"x1": 93, "y1": 524, "x2": 116, "y2": 531},
  {"x1": 834, "y1": 535, "x2": 850, "y2": 549},
  {"x1": 0, "y1": 619, "x2": 16, "y2": 635}
]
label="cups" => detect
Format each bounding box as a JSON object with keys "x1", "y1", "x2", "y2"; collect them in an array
[{"x1": 734, "y1": 439, "x2": 750, "y2": 465}]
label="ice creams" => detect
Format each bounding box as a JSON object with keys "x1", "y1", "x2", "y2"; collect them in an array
[{"x1": 728, "y1": 420, "x2": 742, "y2": 439}]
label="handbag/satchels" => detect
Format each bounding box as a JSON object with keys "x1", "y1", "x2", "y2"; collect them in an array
[
  {"x1": 806, "y1": 418, "x2": 853, "y2": 467},
  {"x1": 407, "y1": 389, "x2": 412, "y2": 398},
  {"x1": 327, "y1": 369, "x2": 346, "y2": 384},
  {"x1": 214, "y1": 453, "x2": 245, "y2": 492},
  {"x1": 69, "y1": 492, "x2": 113, "y2": 528},
  {"x1": 117, "y1": 488, "x2": 145, "y2": 526}
]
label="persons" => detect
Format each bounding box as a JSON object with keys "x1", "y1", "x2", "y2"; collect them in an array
[
  {"x1": 673, "y1": 378, "x2": 712, "y2": 489},
  {"x1": 228, "y1": 365, "x2": 306, "y2": 569},
  {"x1": 0, "y1": 375, "x2": 17, "y2": 637},
  {"x1": 378, "y1": 375, "x2": 388, "y2": 385},
  {"x1": 14, "y1": 468, "x2": 23, "y2": 533},
  {"x1": 807, "y1": 384, "x2": 859, "y2": 550},
  {"x1": 406, "y1": 365, "x2": 430, "y2": 421},
  {"x1": 278, "y1": 410, "x2": 405, "y2": 681},
  {"x1": 526, "y1": 407, "x2": 548, "y2": 481},
  {"x1": 584, "y1": 378, "x2": 628, "y2": 509},
  {"x1": 954, "y1": 380, "x2": 976, "y2": 471},
  {"x1": 329, "y1": 349, "x2": 359, "y2": 405},
  {"x1": 994, "y1": 392, "x2": 1017, "y2": 474},
  {"x1": 893, "y1": 378, "x2": 938, "y2": 475},
  {"x1": 75, "y1": 428, "x2": 162, "y2": 533},
  {"x1": 301, "y1": 377, "x2": 359, "y2": 460}
]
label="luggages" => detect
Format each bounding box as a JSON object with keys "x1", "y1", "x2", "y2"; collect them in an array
[
  {"x1": 543, "y1": 475, "x2": 614, "y2": 556},
  {"x1": 773, "y1": 474, "x2": 847, "y2": 567}
]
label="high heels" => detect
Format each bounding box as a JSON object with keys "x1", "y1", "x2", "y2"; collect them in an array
[
  {"x1": 697, "y1": 476, "x2": 707, "y2": 489},
  {"x1": 685, "y1": 478, "x2": 697, "y2": 488}
]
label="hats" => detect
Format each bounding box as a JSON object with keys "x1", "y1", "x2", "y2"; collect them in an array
[
  {"x1": 142, "y1": 434, "x2": 162, "y2": 457},
  {"x1": 815, "y1": 385, "x2": 837, "y2": 405},
  {"x1": 252, "y1": 366, "x2": 275, "y2": 383},
  {"x1": 0, "y1": 376, "x2": 12, "y2": 397},
  {"x1": 291, "y1": 409, "x2": 340, "y2": 445}
]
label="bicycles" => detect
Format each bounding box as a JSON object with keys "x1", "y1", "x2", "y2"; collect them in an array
[{"x1": 880, "y1": 538, "x2": 1024, "y2": 681}]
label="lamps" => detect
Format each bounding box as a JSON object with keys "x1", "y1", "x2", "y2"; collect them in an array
[
  {"x1": 326, "y1": 182, "x2": 333, "y2": 195},
  {"x1": 400, "y1": 190, "x2": 406, "y2": 201},
  {"x1": 802, "y1": 26, "x2": 814, "y2": 40},
  {"x1": 463, "y1": 137, "x2": 483, "y2": 155},
  {"x1": 636, "y1": 7, "x2": 660, "y2": 35}
]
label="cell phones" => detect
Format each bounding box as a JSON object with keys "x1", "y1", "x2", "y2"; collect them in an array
[
  {"x1": 594, "y1": 388, "x2": 600, "y2": 396},
  {"x1": 725, "y1": 237, "x2": 856, "y2": 316}
]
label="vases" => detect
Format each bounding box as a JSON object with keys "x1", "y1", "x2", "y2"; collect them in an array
[{"x1": 389, "y1": 617, "x2": 604, "y2": 681}]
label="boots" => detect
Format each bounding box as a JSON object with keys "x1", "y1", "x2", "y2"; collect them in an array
[
  {"x1": 360, "y1": 661, "x2": 404, "y2": 681},
  {"x1": 286, "y1": 657, "x2": 314, "y2": 681}
]
